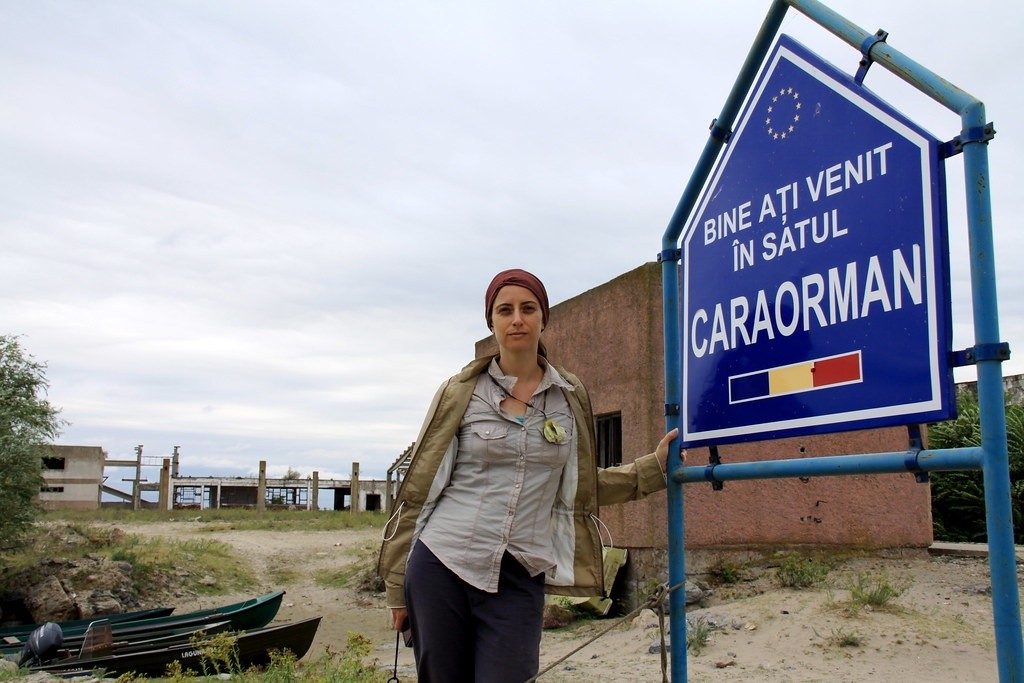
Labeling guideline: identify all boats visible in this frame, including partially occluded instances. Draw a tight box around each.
[0,615,324,678]
[0,607,177,636]
[0,591,286,655]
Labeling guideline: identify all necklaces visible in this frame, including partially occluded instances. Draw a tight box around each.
[484,371,566,444]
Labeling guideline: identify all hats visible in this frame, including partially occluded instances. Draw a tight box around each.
[485,269,550,333]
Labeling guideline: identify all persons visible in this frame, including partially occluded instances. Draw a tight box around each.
[374,269,687,682]
